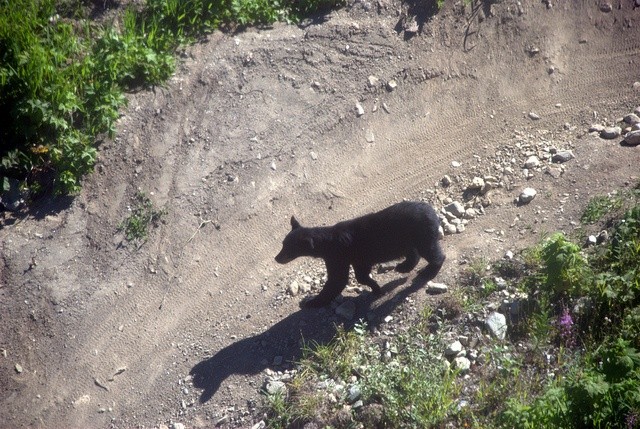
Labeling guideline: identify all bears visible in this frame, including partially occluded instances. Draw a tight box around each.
[274,202,446,307]
